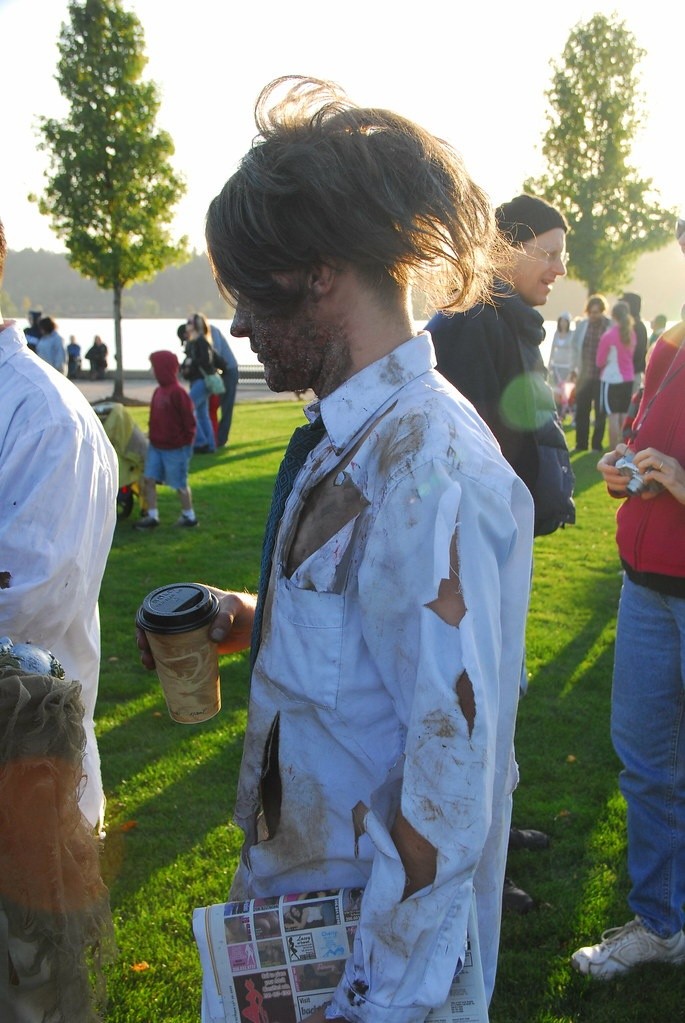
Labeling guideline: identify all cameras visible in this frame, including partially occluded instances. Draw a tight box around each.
[615,454,668,496]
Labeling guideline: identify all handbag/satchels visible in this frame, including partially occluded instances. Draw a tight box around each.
[205,372,227,395]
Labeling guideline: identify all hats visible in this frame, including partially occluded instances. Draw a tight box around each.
[177,325,186,347]
[618,292,642,304]
[494,195,569,246]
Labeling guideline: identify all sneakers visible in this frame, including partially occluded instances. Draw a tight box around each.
[570,915,684,984]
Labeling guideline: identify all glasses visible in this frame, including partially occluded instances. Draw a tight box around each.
[535,251,570,266]
[0,636,65,684]
[675,220,685,240]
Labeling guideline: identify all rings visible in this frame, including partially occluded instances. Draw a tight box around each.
[658,460,664,470]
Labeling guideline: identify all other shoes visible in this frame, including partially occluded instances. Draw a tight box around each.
[171,515,201,529]
[135,516,162,530]
[194,444,216,454]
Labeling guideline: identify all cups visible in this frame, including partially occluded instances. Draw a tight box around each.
[137,581,221,724]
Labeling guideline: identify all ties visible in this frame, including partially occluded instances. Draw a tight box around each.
[250,413,325,691]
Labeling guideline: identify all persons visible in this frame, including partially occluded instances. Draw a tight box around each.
[548,293,685,451]
[569,209,685,979]
[177,311,239,454]
[0,218,119,839]
[136,75,536,1023]
[424,193,577,915]
[348,887,364,911]
[134,350,198,528]
[23,307,107,380]
[284,903,335,929]
